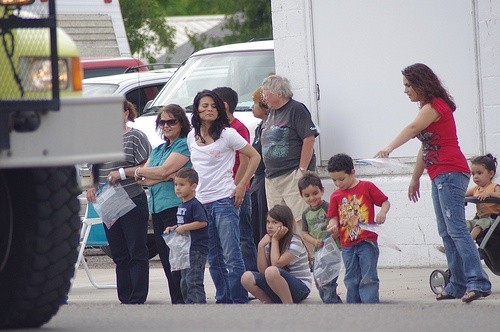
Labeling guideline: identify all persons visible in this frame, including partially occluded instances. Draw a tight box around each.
[260,75,319,263]
[212,87,259,299]
[373,63,492,302]
[241,204,312,304]
[87,100,152,304]
[327,153,390,303]
[437,154,500,253]
[187,90,261,304]
[134,104,193,304]
[250,88,268,252]
[163,168,208,304]
[298,171,344,304]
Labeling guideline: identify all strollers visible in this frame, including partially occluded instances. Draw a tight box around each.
[429,197,500,295]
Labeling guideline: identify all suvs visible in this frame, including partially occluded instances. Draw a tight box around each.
[0,0,127,330]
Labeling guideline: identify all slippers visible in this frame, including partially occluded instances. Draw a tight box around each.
[462,290,489,303]
[436,292,456,300]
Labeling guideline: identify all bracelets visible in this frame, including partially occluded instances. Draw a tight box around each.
[118,168,127,180]
[299,167,306,173]
[135,167,142,178]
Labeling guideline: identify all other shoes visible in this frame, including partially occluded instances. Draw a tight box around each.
[438,245,445,253]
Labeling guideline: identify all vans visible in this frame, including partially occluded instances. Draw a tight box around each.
[78,38,274,259]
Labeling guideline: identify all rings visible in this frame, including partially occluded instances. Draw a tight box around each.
[108,176,112,182]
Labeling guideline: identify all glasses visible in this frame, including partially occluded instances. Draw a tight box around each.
[159,118,177,126]
